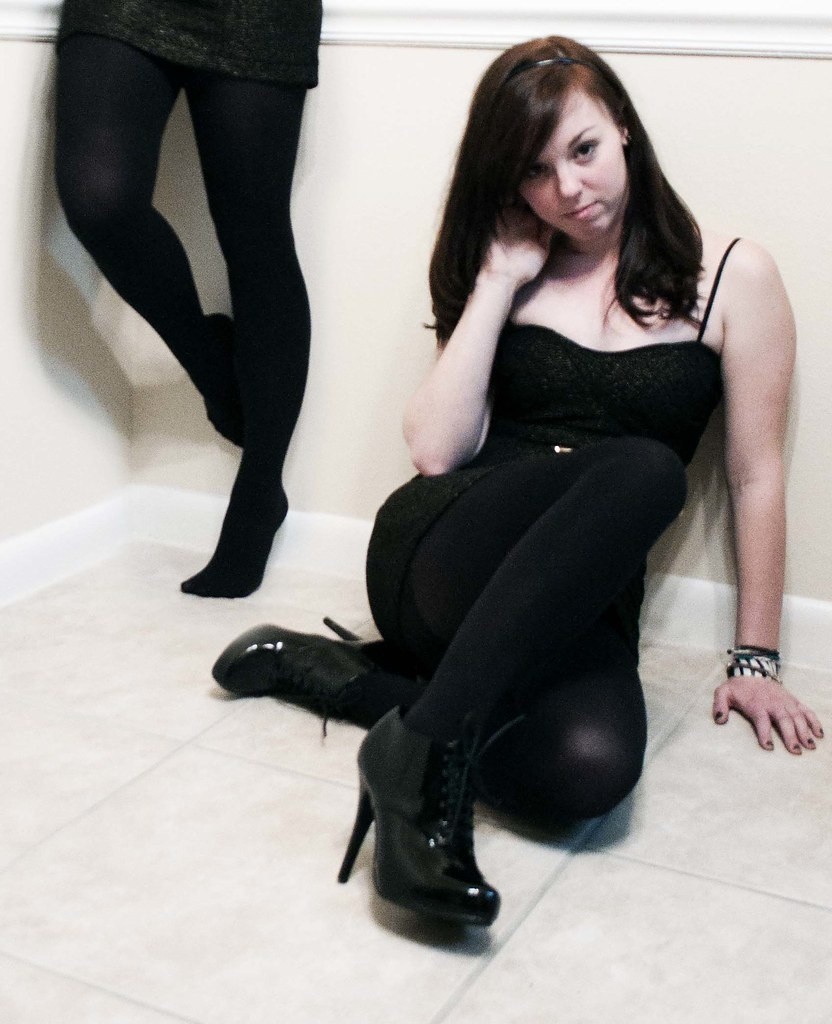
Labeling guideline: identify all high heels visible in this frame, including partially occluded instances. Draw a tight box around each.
[336,706,504,928]
[213,618,390,723]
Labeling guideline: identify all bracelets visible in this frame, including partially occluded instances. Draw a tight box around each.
[726,644,780,682]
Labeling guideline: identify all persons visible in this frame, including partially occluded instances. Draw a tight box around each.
[52,0,324,599]
[211,37,823,929]
